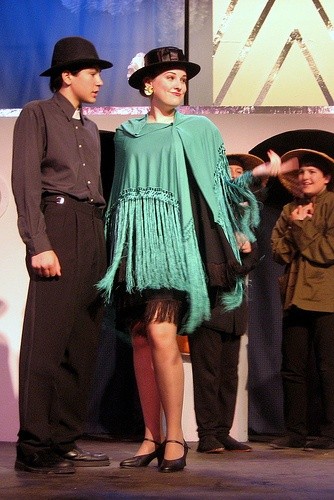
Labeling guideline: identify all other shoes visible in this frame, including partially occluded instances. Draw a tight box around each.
[196,435,224,453]
[301,437,334,451]
[268,434,302,449]
[217,434,253,452]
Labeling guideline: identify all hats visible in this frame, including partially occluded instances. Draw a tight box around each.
[278,149,334,198]
[226,153,269,194]
[131,44,203,90]
[41,37,113,74]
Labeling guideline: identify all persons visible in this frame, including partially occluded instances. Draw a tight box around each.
[95,46,334,473]
[11,37,112,473]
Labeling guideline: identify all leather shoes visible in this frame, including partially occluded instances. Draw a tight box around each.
[14,460,78,478]
[62,446,110,467]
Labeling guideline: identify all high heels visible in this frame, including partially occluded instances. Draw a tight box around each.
[120,437,166,469]
[158,439,191,473]
